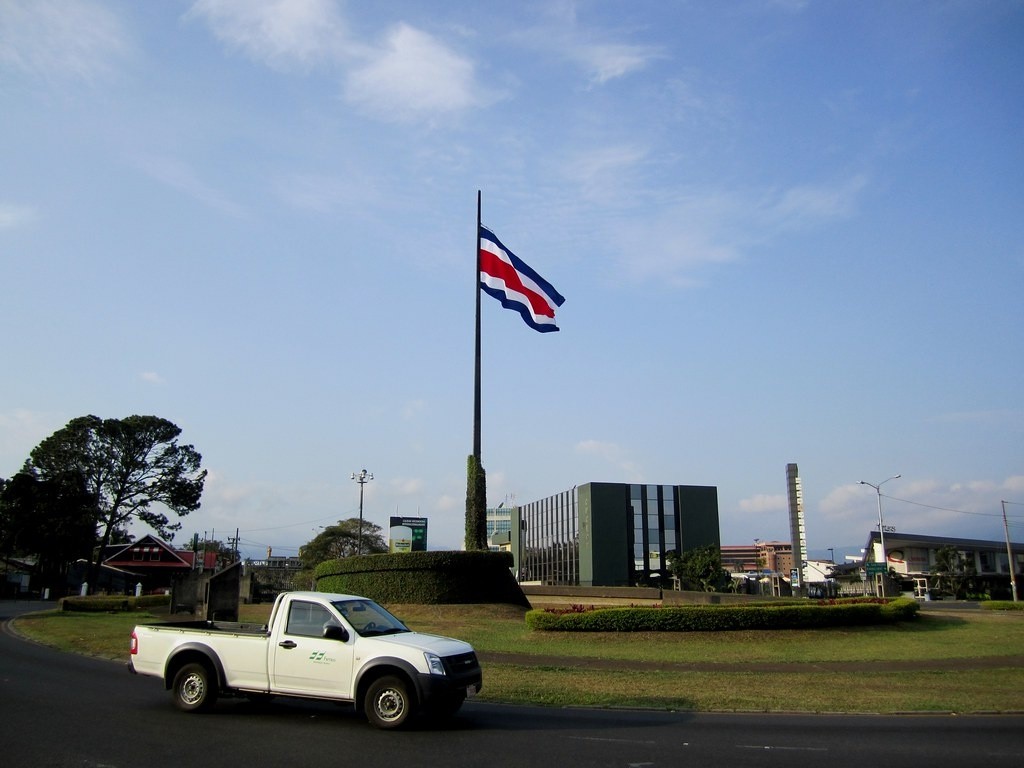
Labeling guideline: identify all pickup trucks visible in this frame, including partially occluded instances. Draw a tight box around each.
[128,591,482,731]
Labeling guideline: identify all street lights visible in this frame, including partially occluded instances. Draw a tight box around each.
[754,539,759,574]
[351,469,374,554]
[4,543,17,580]
[856,474,904,562]
[828,548,834,565]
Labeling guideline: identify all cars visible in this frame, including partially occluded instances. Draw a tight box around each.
[143,584,170,595]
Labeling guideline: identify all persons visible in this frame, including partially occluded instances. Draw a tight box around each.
[322,604,348,637]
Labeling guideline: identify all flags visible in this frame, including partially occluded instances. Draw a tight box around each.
[478,222,567,334]
